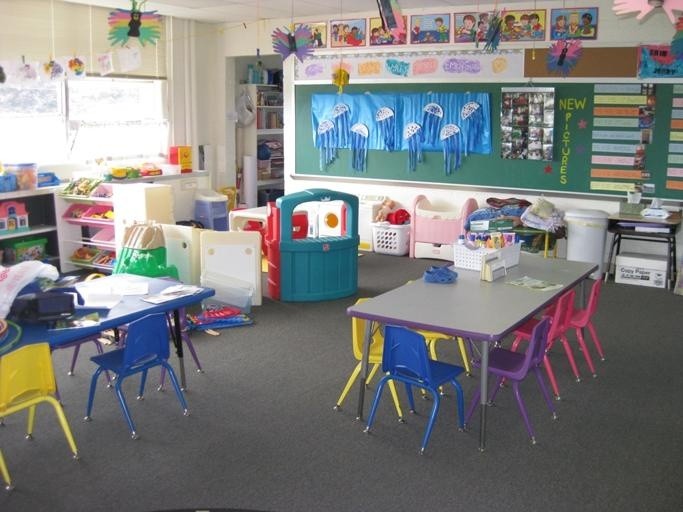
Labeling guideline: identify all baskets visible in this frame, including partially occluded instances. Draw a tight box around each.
[369,220,411,258]
[453,239,525,273]
[61,181,115,274]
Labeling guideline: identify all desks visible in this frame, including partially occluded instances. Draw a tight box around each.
[604,212,681,293]
[347,250,598,454]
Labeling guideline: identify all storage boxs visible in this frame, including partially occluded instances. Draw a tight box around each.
[615,251,669,288]
[62,178,116,269]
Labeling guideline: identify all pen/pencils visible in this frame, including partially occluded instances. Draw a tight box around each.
[458,234,512,249]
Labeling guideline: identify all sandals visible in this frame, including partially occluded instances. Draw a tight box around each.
[425,265,458,284]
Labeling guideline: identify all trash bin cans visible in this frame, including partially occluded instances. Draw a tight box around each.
[563,209,609,280]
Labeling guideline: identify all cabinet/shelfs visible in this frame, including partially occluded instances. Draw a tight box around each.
[157,172,213,222]
[194,192,228,231]
[234,83,283,207]
[58,182,172,277]
[409,196,475,261]
[0,181,81,273]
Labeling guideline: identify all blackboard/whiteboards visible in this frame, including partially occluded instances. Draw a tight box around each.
[290,82,683,206]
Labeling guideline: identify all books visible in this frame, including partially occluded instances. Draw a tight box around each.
[257,108,284,181]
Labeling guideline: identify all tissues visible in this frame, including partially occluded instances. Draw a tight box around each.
[641,198,672,219]
[619,192,645,218]
